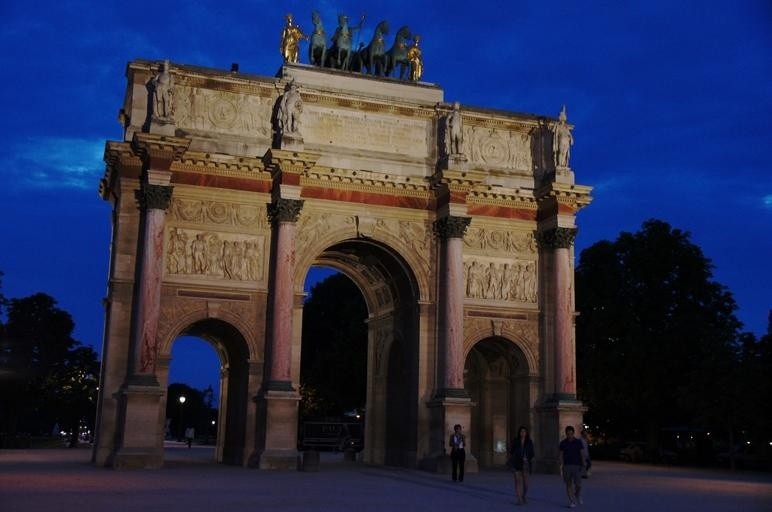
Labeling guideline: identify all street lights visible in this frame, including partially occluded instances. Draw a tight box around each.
[202,384,213,444]
[175,392,187,438]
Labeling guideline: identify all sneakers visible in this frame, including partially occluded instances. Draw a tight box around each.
[569,494,584,508]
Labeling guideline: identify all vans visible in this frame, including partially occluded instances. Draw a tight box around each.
[301,420,364,451]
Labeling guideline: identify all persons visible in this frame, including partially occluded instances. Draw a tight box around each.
[449,424,466,483]
[277,84,304,133]
[579,428,591,479]
[408,37,424,81]
[446,109,464,154]
[552,124,574,168]
[185,424,195,448]
[465,257,539,303]
[153,59,174,117]
[508,425,534,505]
[280,16,303,65]
[558,425,586,507]
[162,228,263,281]
